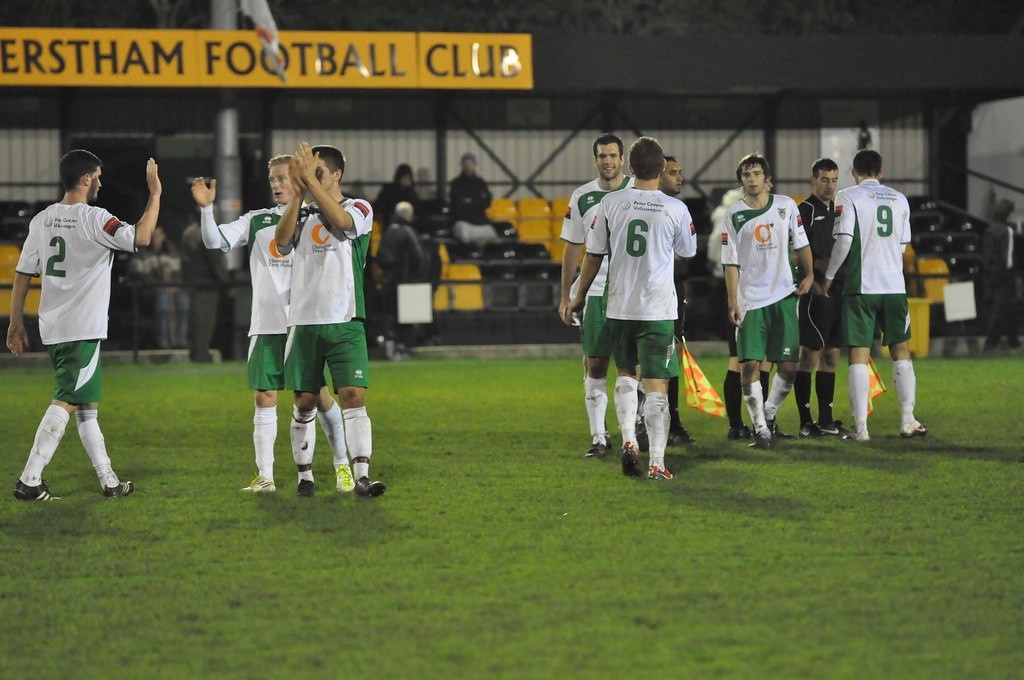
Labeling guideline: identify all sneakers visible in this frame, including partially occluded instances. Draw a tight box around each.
[103,478,134,499]
[354,476,386,497]
[900,420,928,438]
[799,420,851,440]
[841,429,871,441]
[334,464,355,493]
[648,466,676,481]
[13,478,62,501]
[616,441,643,478]
[727,425,754,439]
[240,472,276,495]
[768,423,796,440]
[748,425,772,450]
[297,479,315,497]
[636,426,697,451]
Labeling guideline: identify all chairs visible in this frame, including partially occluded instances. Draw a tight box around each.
[0,196,1024,319]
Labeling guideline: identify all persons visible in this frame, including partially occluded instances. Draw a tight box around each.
[362,153,493,361]
[558,134,928,478]
[192,155,355,492]
[981,198,1024,353]
[7,150,163,501]
[274,142,386,497]
[130,204,238,362]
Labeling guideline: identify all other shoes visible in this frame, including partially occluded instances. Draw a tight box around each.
[585,436,611,459]
[377,334,413,362]
